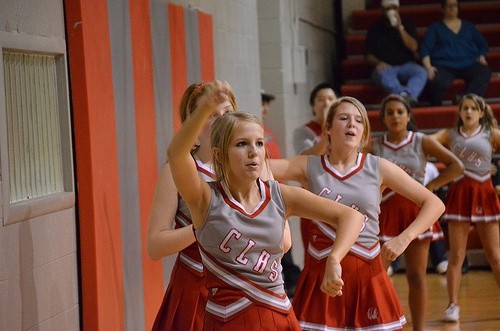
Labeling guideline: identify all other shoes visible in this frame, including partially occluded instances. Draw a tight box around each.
[435,260,449,274]
[401,91,418,105]
[387,265,398,277]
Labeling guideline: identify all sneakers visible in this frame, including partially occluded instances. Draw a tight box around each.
[445,303,461,322]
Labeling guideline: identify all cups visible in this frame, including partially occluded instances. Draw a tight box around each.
[387,9,398,26]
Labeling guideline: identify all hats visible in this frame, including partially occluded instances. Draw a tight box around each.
[381,0,399,8]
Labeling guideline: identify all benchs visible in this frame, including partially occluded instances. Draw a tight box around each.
[341,2,500,273]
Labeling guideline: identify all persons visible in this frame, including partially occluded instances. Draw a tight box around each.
[148,83,292,331]
[265,97,446,331]
[166,79,364,331]
[261,0,500,331]
